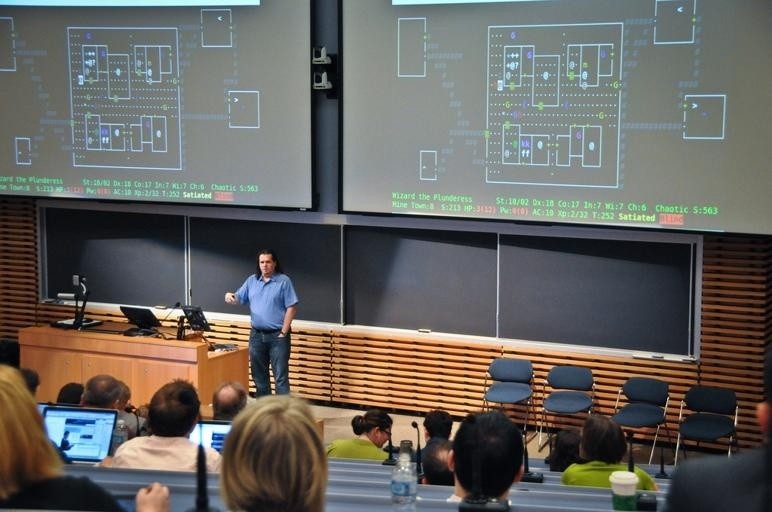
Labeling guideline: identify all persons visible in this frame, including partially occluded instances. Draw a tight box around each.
[18,369,146,456]
[561,416,659,490]
[225,248,299,396]
[326,409,393,461]
[220,395,328,512]
[447,411,525,512]
[661,348,772,512]
[111,378,222,474]
[549,429,589,471]
[212,382,248,420]
[61,431,71,450]
[0,363,171,512]
[420,437,455,486]
[411,409,452,462]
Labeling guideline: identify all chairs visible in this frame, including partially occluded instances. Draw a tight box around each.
[479,356,542,448]
[611,374,677,466]
[674,383,741,464]
[536,361,599,452]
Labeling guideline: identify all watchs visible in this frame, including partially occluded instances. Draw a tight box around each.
[280,331,288,337]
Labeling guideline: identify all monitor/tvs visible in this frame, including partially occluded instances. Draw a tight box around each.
[183,305,211,332]
[120,306,162,329]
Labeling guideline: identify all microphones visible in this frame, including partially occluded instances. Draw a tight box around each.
[156,302,180,331]
[125,407,140,437]
[412,421,424,480]
[519,431,543,482]
[626,429,657,512]
[382,432,398,466]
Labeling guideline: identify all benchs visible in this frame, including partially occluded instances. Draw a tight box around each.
[323,454,457,509]
[507,458,675,512]
[65,461,222,509]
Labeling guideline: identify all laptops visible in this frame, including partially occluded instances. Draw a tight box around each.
[37,402,80,418]
[42,406,118,467]
[189,420,232,458]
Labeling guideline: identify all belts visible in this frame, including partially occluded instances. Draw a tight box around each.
[251,328,280,334]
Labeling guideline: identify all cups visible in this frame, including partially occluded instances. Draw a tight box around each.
[639,492,656,512]
[608,469,640,512]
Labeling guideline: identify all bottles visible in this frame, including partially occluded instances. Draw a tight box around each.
[390,438,420,511]
